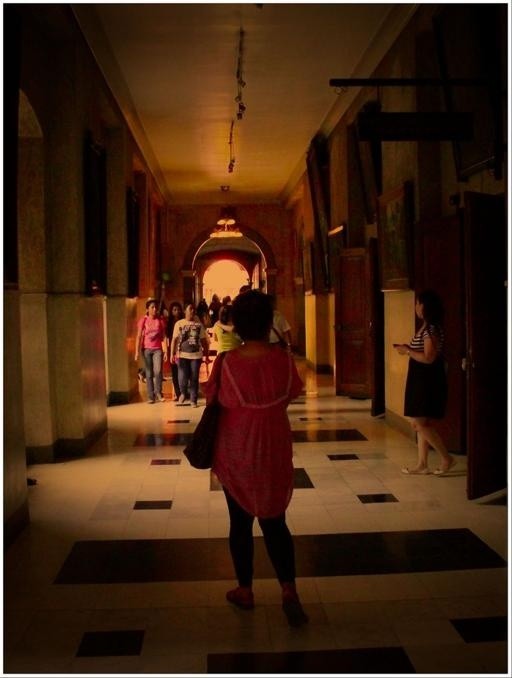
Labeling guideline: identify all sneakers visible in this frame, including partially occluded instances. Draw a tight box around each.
[173,393,185,405]
[191,402,197,408]
[147,394,166,403]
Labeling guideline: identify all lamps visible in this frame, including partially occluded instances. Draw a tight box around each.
[210,186,243,238]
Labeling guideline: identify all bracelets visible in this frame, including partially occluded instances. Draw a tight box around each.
[406,348,412,358]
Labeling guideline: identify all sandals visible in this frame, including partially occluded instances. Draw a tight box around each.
[434,456,459,477]
[403,466,430,476]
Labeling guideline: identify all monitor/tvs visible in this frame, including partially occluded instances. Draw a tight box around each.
[303,243,313,296]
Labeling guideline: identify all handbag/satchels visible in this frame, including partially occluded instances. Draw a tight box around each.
[182,349,230,470]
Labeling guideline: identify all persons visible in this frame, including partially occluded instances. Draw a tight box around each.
[204,288,314,630]
[134,282,300,408]
[390,278,458,478]
